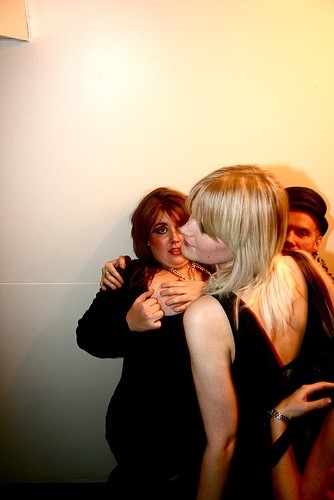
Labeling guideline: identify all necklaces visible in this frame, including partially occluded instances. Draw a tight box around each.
[160,261,198,281]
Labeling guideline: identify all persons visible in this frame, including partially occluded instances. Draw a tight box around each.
[76,187,219,500]
[281,187,328,256]
[269,381,334,500]
[178,164,334,500]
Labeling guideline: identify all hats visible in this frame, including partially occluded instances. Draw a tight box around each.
[283,186,329,237]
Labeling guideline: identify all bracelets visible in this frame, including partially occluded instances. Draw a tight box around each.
[270,409,291,425]
